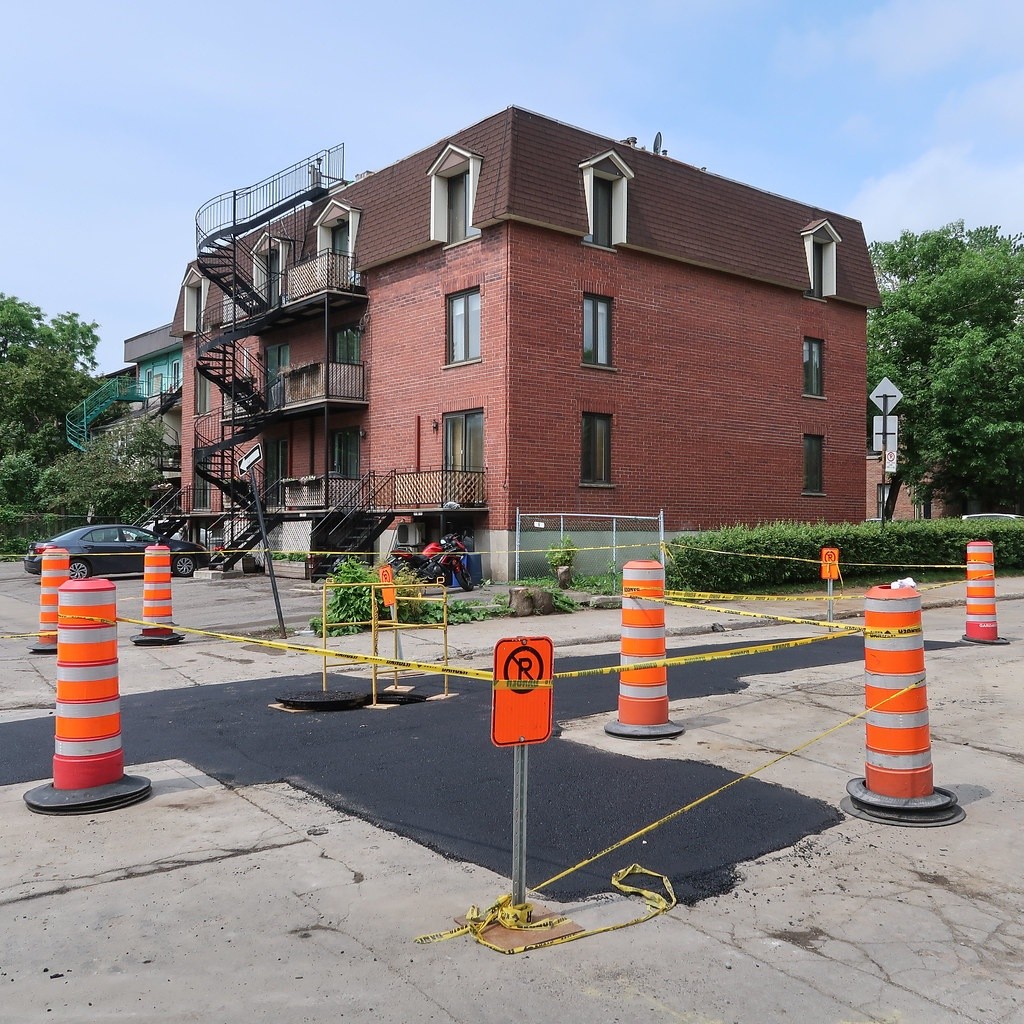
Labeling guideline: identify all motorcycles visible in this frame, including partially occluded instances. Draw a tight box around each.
[385,531,474,594]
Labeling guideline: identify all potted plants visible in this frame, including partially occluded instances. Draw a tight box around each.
[264,553,315,579]
[277,360,318,378]
[544,535,578,590]
[280,478,300,487]
[298,475,319,486]
[234,554,256,573]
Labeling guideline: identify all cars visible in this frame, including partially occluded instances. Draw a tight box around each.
[24,524,209,581]
[115,519,212,547]
[866,518,893,524]
[961,512,1024,525]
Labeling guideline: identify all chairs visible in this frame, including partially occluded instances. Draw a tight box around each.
[105,533,142,542]
